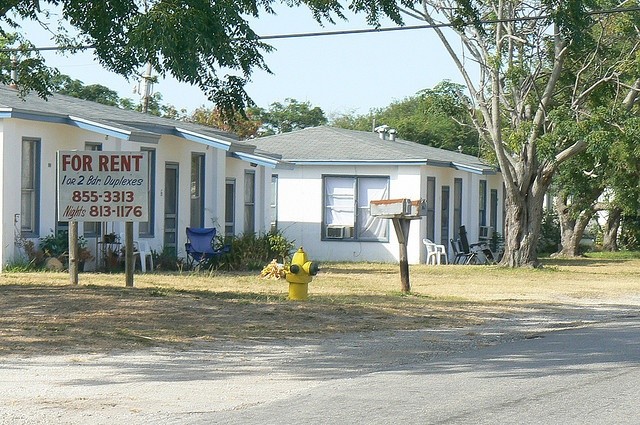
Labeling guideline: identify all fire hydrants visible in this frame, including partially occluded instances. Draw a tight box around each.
[285,246,319,302]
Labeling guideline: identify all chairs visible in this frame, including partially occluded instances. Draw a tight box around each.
[450,239,478,265]
[456,239,482,265]
[423,238,449,266]
[458,225,498,265]
[185,227,231,271]
[132,241,154,275]
